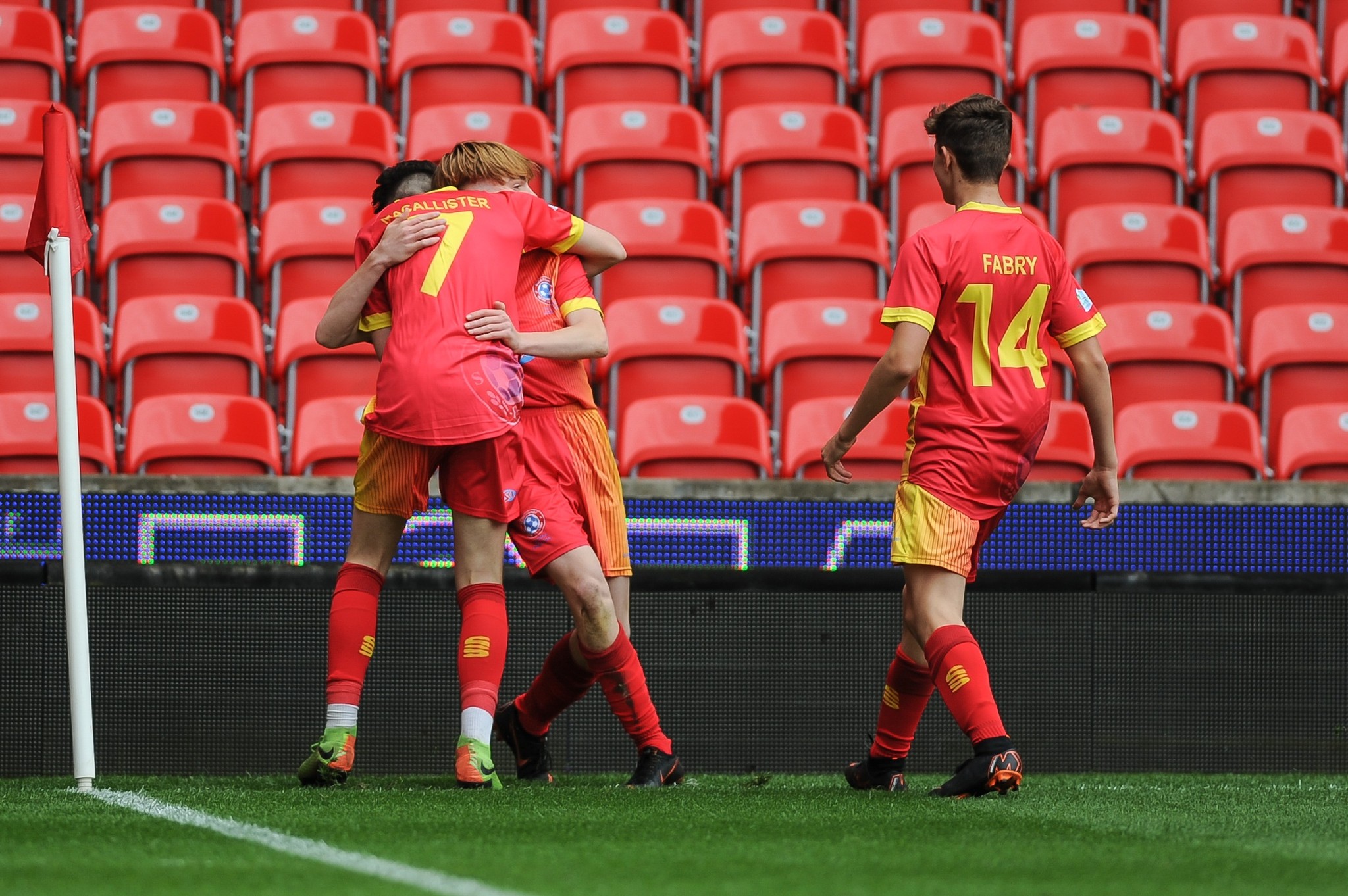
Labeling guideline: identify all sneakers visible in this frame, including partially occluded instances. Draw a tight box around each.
[455,736,503,790]
[296,725,357,787]
[627,746,685,790]
[491,700,553,784]
[927,747,1023,800]
[844,758,905,794]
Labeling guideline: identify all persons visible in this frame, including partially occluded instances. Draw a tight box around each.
[315,141,687,790]
[821,94,1120,795]
[296,160,602,790]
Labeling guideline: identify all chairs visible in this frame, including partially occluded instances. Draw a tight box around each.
[0,0,1348,482]
